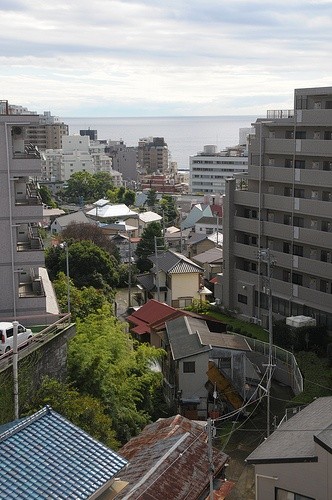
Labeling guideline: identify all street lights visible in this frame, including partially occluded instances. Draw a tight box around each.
[60,241,71,314]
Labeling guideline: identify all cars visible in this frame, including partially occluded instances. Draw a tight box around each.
[0,321,32,356]
